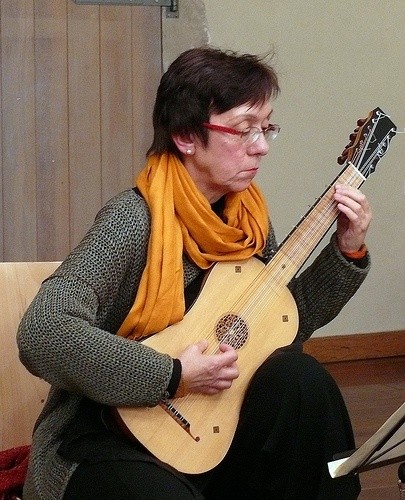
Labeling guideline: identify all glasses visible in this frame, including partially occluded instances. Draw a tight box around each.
[199,123,280,145]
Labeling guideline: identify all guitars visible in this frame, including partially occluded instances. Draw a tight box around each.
[115,106,397,483]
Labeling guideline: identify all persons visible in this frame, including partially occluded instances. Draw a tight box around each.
[16,48,374,500]
